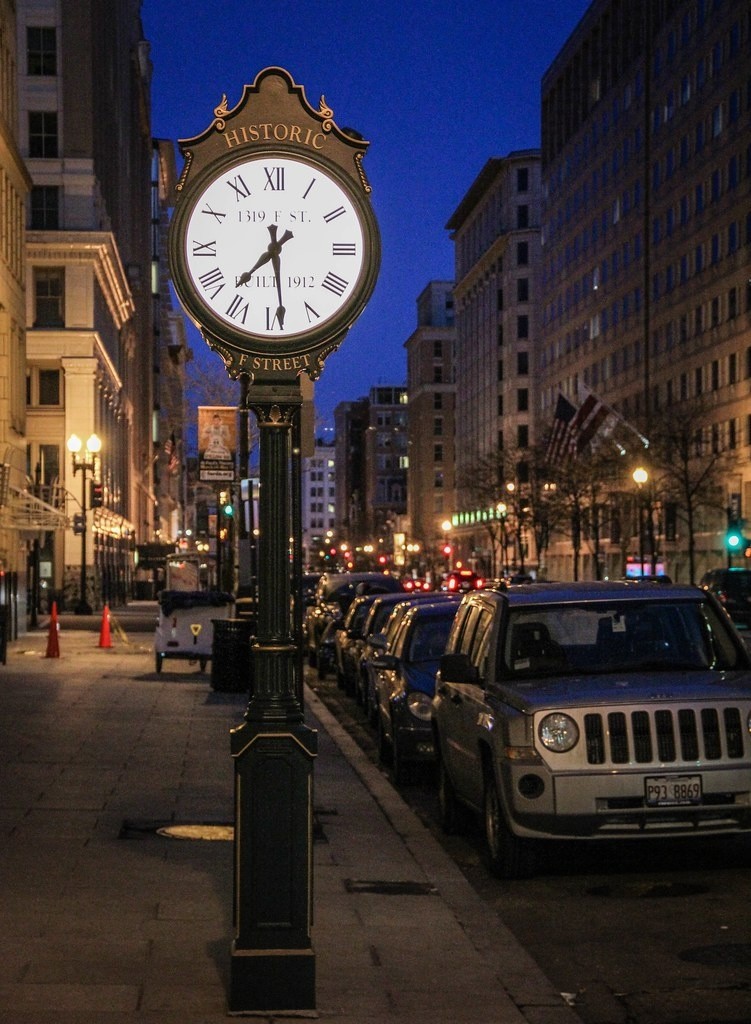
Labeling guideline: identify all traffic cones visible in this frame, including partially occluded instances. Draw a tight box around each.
[43,603,61,658]
[96,604,115,648]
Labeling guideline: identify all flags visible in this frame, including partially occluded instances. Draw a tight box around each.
[545,387,618,470]
[163,433,180,468]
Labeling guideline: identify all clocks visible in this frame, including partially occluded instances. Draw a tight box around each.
[167,66,380,405]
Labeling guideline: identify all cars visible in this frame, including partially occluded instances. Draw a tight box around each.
[318,592,464,722]
[442,571,485,596]
[405,576,431,592]
[370,599,461,789]
[290,571,327,629]
[304,573,400,666]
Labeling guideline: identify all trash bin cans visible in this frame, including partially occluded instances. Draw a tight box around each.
[210,617,253,693]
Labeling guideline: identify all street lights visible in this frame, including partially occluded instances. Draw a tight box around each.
[496,501,510,581]
[67,433,103,615]
[442,521,450,571]
[633,466,648,576]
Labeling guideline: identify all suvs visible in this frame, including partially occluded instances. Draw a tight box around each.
[701,567,751,646]
[431,578,751,878]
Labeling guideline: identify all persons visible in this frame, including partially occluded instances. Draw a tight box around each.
[156,568,165,590]
[201,415,232,446]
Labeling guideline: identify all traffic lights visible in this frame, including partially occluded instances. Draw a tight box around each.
[90,479,104,508]
[224,505,233,515]
[727,533,743,549]
